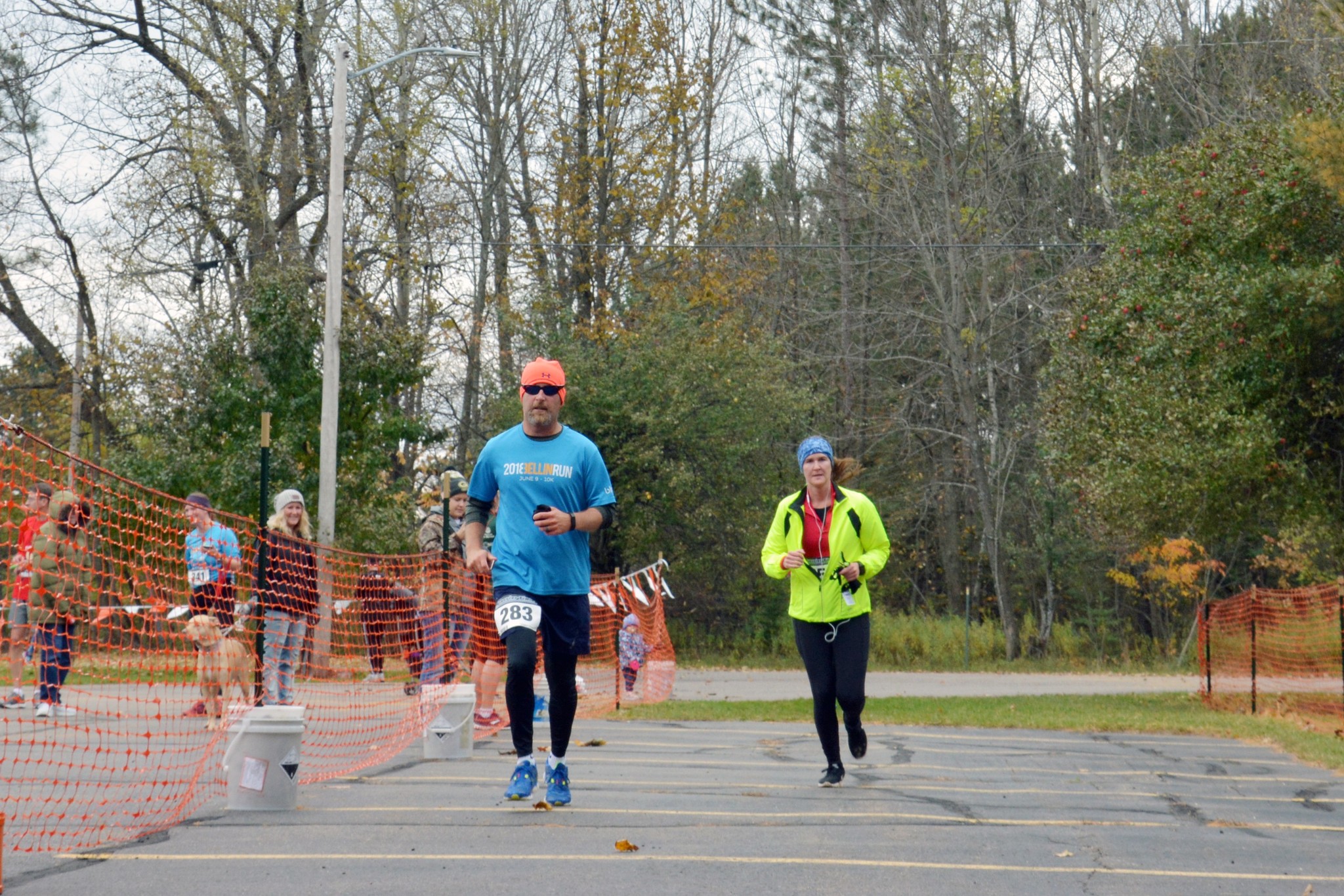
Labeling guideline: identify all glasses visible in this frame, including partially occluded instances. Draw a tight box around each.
[521,384,564,396]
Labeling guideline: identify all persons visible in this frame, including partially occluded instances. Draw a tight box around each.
[168,491,241,718]
[760,435,890,788]
[0,482,54,711]
[616,613,654,702]
[354,469,510,734]
[247,489,322,708]
[463,356,616,806]
[27,490,92,718]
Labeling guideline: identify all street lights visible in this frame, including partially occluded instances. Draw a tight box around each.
[307,40,481,680]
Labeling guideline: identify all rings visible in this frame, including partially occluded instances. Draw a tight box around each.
[546,526,550,533]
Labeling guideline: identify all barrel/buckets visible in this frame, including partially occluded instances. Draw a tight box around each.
[417,683,477,760]
[221,704,305,811]
[532,673,550,728]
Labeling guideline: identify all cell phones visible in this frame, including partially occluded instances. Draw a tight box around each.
[488,559,495,570]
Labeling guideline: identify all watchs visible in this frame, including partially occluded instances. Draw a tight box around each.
[854,561,866,576]
[568,512,576,532]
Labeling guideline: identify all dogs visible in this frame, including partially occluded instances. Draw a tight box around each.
[180,614,250,730]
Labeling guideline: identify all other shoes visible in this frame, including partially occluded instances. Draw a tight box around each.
[364,672,383,685]
[54,703,75,717]
[36,706,50,717]
[625,693,641,701]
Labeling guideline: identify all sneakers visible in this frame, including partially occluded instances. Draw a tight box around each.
[505,760,538,800]
[844,713,867,760]
[184,699,224,717]
[474,710,510,729]
[0,695,24,708]
[544,755,570,806]
[817,766,845,787]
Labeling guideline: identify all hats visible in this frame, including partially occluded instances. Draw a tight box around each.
[273,489,304,512]
[520,359,566,406]
[186,492,210,508]
[440,467,468,501]
[623,613,640,629]
